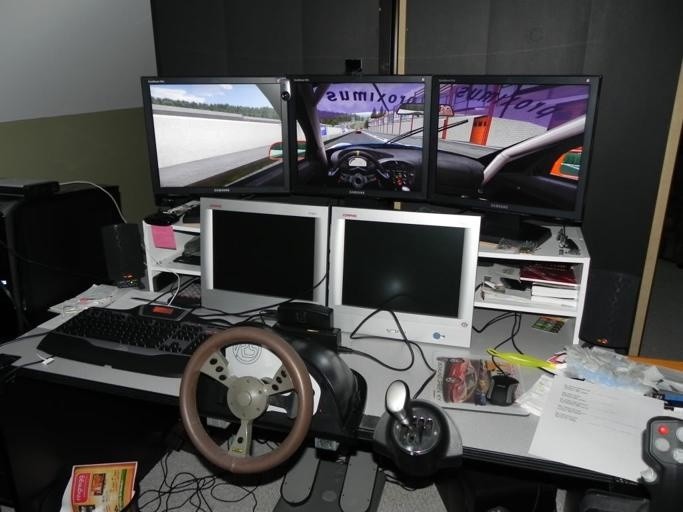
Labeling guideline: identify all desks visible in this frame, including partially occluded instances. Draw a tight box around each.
[0,282,683,511]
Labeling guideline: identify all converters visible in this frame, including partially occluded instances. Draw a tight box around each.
[2,173,61,199]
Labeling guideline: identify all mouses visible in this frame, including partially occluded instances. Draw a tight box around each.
[485,372,520,407]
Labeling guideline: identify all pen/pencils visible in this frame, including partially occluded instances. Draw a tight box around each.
[130,298,168,305]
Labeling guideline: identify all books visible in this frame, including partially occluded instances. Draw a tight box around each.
[481,261,578,309]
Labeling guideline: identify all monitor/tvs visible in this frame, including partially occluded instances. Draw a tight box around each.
[137,73,292,225]
[434,71,603,252]
[328,203,483,351]
[294,69,431,209]
[199,194,329,320]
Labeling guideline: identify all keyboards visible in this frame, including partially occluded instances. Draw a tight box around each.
[169,279,199,303]
[37,307,219,378]
